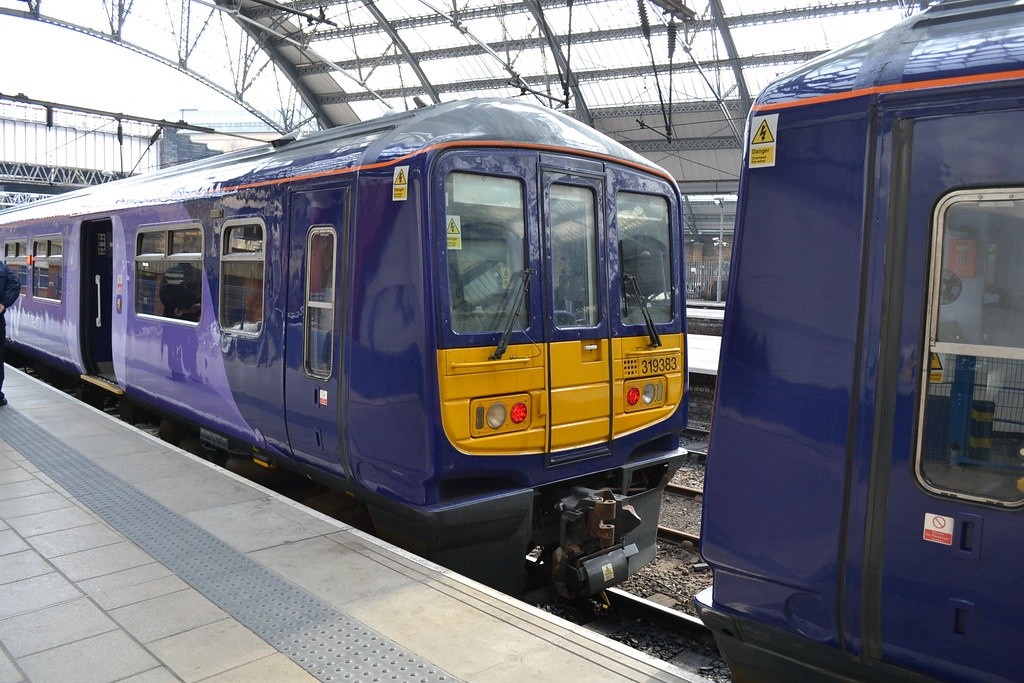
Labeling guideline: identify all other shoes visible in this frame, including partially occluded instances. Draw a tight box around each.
[0,392,7,406]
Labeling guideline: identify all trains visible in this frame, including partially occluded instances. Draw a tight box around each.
[0,94,688,599]
[688,1,1023,683]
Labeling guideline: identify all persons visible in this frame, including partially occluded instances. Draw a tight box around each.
[159,251,204,383]
[0,260,21,406]
